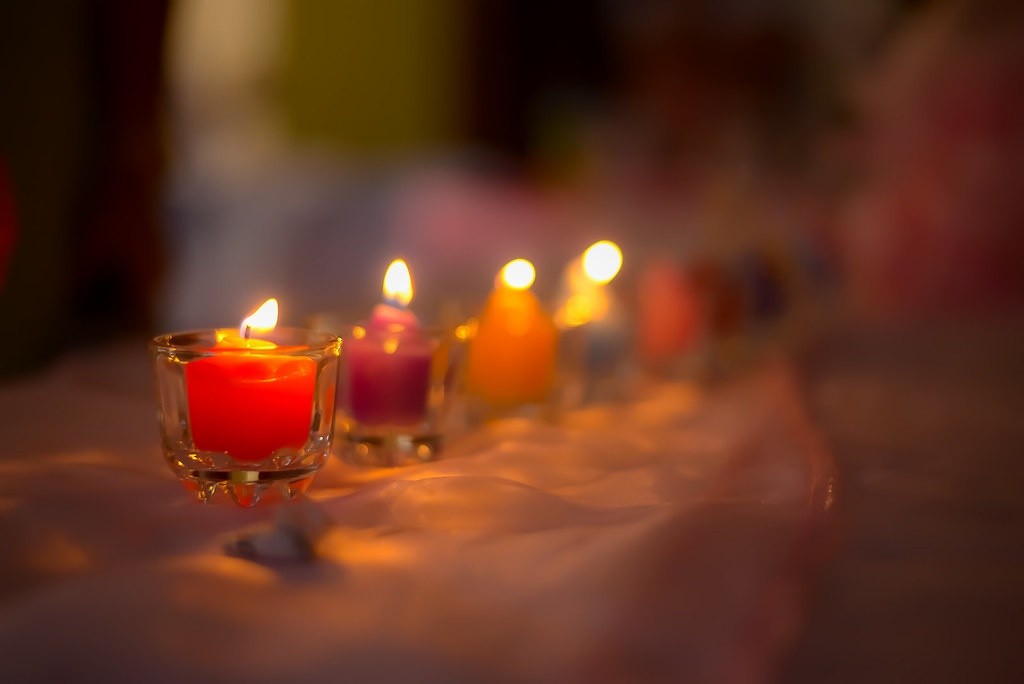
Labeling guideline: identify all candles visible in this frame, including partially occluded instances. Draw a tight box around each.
[343,259,437,427]
[555,240,636,377]
[183,298,319,467]
[466,256,556,406]
